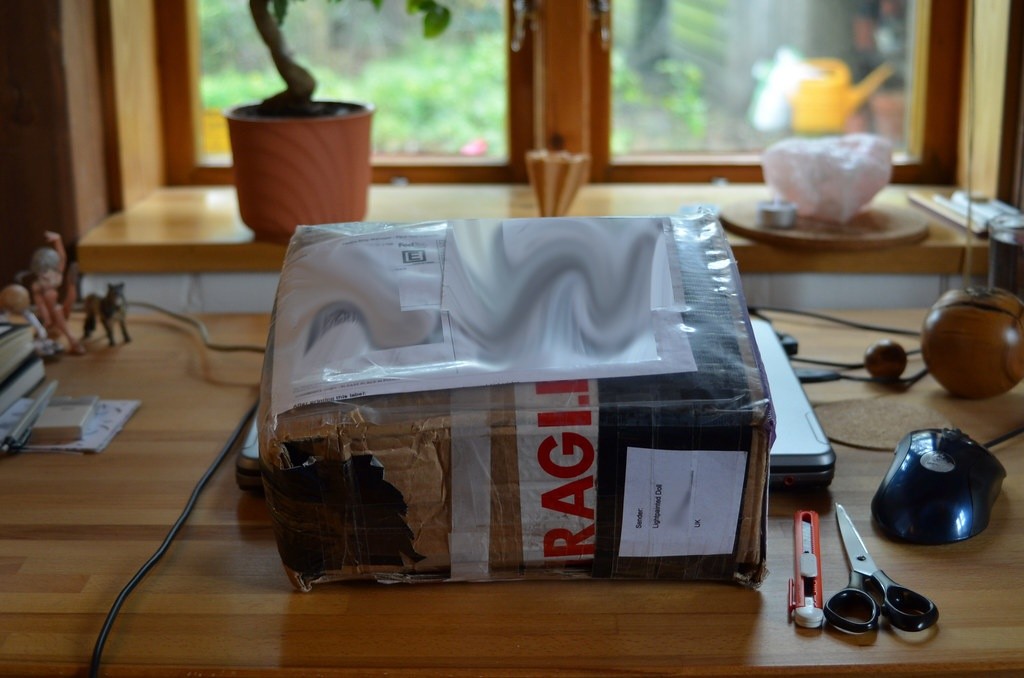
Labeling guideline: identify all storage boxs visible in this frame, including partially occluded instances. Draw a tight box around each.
[259,210,782,593]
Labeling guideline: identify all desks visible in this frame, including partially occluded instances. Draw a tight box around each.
[0,302,1024,677]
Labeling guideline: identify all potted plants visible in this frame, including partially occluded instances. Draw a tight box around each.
[222,1,451,243]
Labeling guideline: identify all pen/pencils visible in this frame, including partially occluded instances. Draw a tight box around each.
[0,379,63,455]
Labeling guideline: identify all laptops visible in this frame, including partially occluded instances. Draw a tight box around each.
[232,319,836,496]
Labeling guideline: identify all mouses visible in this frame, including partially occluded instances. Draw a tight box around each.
[871,427,1007,544]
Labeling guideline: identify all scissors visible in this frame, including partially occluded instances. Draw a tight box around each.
[824,499,939,638]
